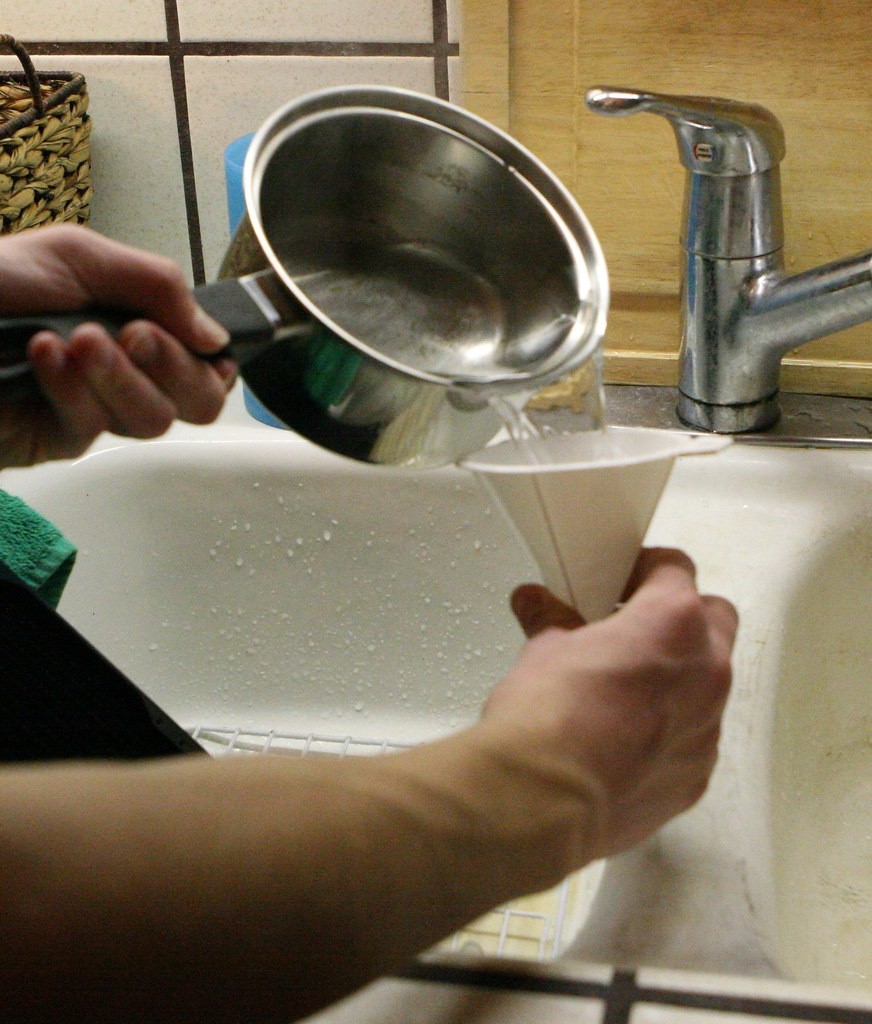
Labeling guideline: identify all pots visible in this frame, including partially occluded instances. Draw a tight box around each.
[0,85,609,470]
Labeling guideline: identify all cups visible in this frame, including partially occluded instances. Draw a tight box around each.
[224,132,291,431]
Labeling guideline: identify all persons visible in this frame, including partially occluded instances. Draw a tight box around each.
[0,223,739,1024]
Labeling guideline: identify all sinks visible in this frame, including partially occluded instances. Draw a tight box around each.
[709,428,871,1023]
[1,405,694,972]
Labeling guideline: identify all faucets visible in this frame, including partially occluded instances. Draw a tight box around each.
[582,84,871,435]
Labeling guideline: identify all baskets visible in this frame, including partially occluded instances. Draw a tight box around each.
[0,32,93,235]
